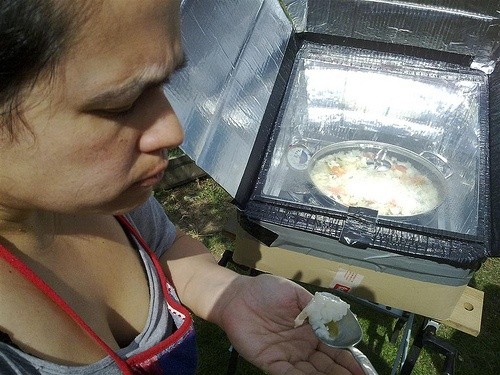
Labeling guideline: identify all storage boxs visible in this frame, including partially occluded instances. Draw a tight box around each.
[166,1,487,278]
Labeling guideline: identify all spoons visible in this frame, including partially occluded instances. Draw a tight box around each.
[309,292,377,375]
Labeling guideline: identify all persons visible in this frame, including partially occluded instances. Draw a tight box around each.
[0,0,367,375]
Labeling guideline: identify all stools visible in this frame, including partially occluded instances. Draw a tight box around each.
[222,211,485,375]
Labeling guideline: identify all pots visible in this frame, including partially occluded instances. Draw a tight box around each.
[285,140,455,225]
